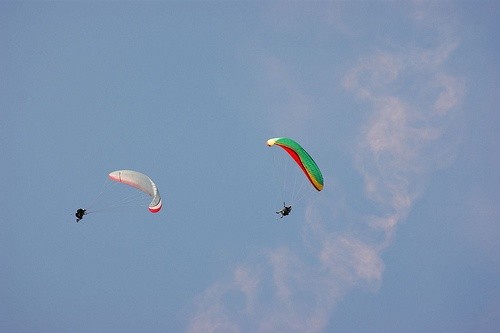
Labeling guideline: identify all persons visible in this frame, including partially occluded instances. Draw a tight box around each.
[75,208,86,222]
[276,201,291,218]
